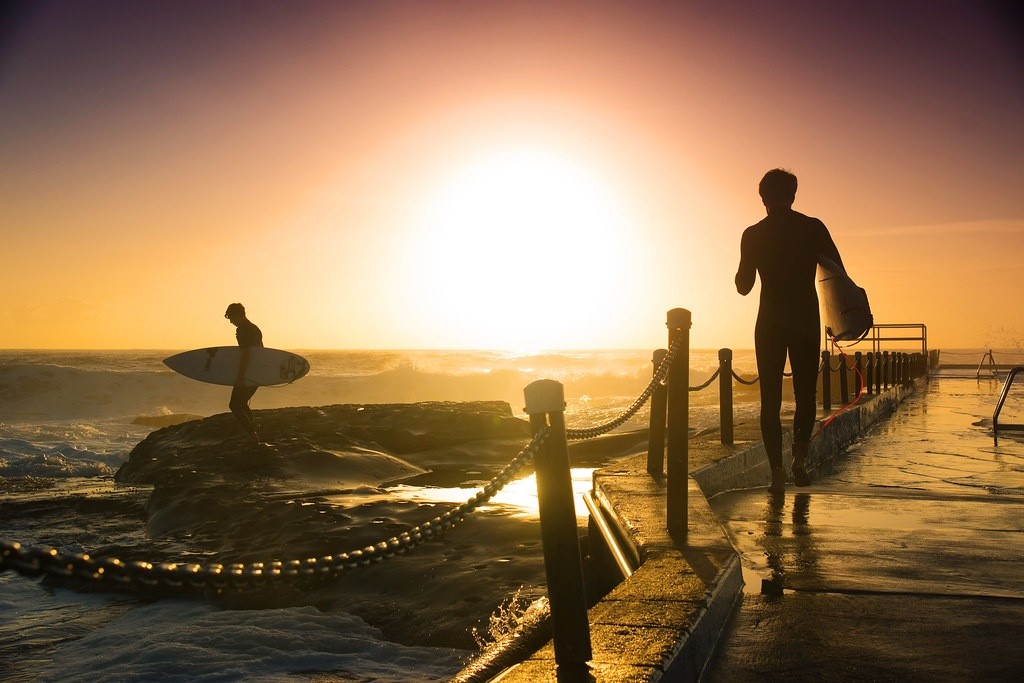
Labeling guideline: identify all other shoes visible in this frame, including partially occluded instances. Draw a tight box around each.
[768,481,785,493]
[792,458,812,488]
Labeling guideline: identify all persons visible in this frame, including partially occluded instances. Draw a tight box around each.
[225,303,264,444]
[734,168,846,494]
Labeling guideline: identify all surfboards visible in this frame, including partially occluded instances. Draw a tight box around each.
[162,345,310,390]
[815,249,874,342]
